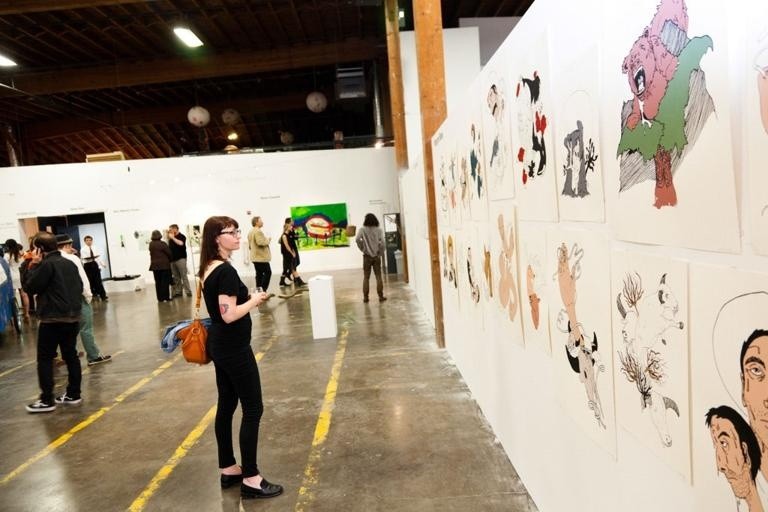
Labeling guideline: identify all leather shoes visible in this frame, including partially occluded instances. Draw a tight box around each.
[239,477,285,499]
[218,465,244,489]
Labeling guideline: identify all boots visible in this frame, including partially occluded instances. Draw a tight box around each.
[294,276,308,286]
[279,276,292,287]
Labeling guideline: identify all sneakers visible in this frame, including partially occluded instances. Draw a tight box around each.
[24,399,56,413]
[155,290,195,303]
[86,353,113,366]
[53,393,82,404]
[363,293,370,303]
[285,272,293,281]
[377,291,387,302]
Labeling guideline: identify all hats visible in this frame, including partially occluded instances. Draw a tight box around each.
[150,230,162,239]
[55,233,73,246]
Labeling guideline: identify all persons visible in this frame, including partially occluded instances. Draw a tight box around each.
[285,218,293,281]
[0,231,113,414]
[167,224,192,297]
[247,215,272,292]
[704,405,765,511]
[355,212,387,302]
[148,230,173,302]
[739,329,768,484]
[192,215,285,499]
[279,223,308,286]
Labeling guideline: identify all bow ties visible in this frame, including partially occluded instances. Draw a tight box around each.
[89,247,95,262]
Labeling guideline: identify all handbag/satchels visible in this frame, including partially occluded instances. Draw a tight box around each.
[177,276,214,365]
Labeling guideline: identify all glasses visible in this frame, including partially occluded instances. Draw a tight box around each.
[219,228,243,239]
